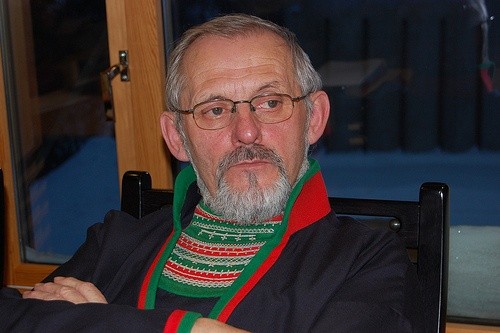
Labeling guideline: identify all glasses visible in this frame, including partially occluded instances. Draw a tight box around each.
[174,86,314,130]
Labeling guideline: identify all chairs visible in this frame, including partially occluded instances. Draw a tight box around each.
[120,169,449,331]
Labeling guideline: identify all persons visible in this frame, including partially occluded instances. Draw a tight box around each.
[444,0,491,148]
[1,13,416,332]
